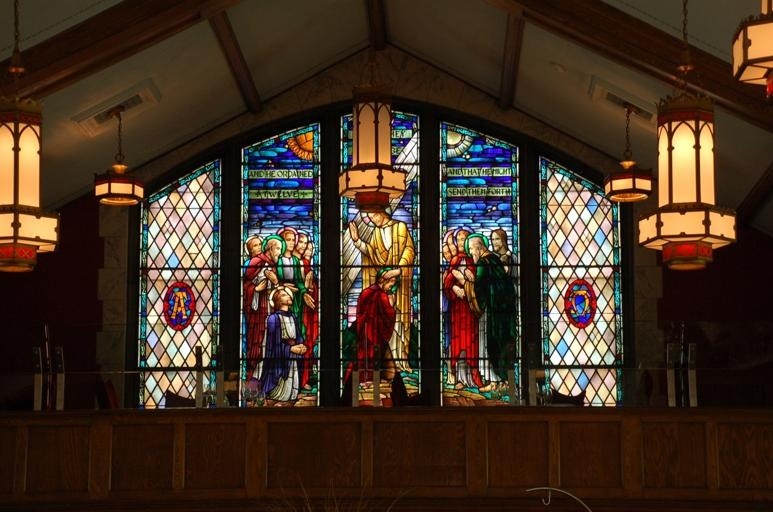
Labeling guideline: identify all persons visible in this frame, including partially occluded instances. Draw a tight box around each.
[442,229,518,392]
[349,207,416,381]
[244,226,318,401]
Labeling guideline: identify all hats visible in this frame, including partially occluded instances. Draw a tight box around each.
[355,192,390,213]
[269,287,293,308]
[244,227,315,258]
[443,227,488,258]
[376,268,401,295]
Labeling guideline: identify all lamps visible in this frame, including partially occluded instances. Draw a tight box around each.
[730,1,772,99]
[0,0,60,274]
[338,41,408,211]
[639,2,739,271]
[604,105,654,206]
[93,107,146,208]
